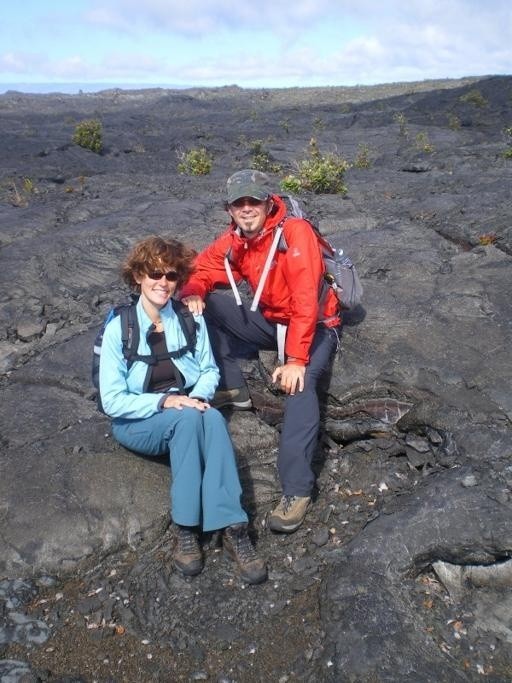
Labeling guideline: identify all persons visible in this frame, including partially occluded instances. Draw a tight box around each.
[178,169,343,532]
[96,236,268,585]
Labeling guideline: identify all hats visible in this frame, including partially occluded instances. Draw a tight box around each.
[226,169,272,206]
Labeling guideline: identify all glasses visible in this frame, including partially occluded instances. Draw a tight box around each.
[145,268,180,281]
[232,198,261,206]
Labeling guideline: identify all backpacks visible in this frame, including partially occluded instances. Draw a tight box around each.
[92,301,197,389]
[274,213,364,310]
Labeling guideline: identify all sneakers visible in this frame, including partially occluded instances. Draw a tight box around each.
[210,387,252,408]
[172,521,203,575]
[223,522,266,584]
[270,494,313,533]
